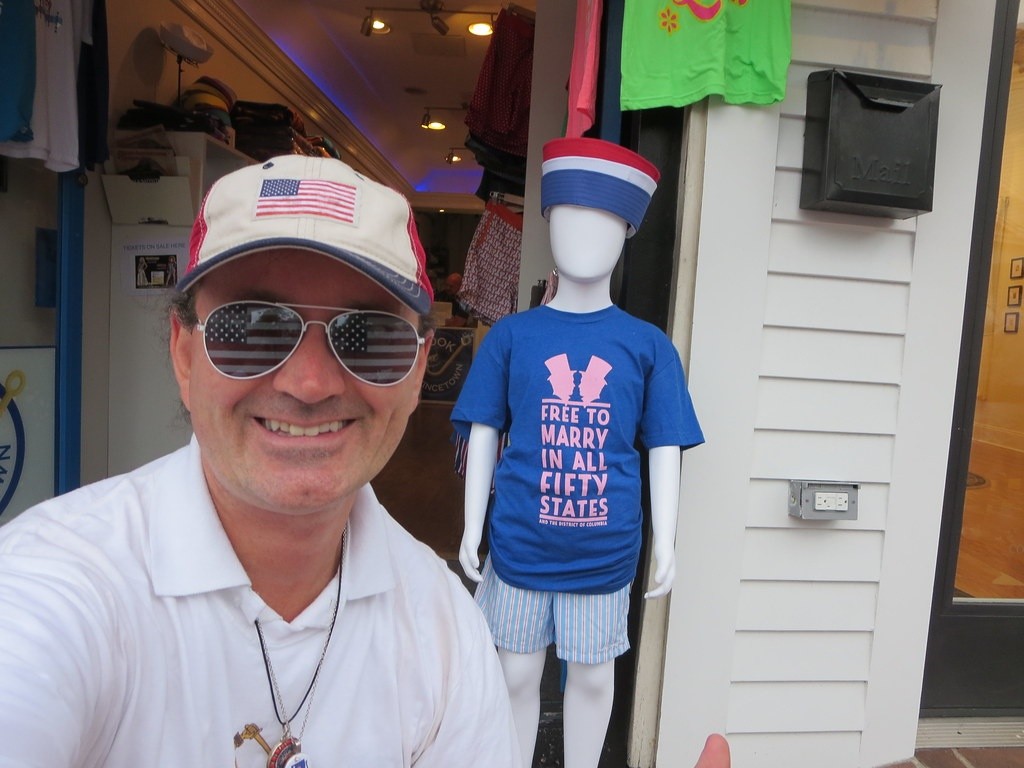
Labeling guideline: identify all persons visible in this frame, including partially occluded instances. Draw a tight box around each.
[449,137,706,768]
[0,154,731,768]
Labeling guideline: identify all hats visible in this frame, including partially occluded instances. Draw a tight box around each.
[541,135,660,237]
[160,20,214,63]
[179,77,237,126]
[175,155,435,313]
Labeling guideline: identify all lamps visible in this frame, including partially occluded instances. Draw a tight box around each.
[446,147,470,165]
[360,6,498,37]
[422,106,432,129]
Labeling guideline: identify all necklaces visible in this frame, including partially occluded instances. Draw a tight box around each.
[253,528,346,768]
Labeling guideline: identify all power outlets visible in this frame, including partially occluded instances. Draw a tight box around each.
[814,492,848,511]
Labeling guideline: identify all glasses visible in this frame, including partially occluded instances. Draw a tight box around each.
[197,300,427,387]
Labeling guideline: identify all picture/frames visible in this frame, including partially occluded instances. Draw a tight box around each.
[1003,256,1024,333]
